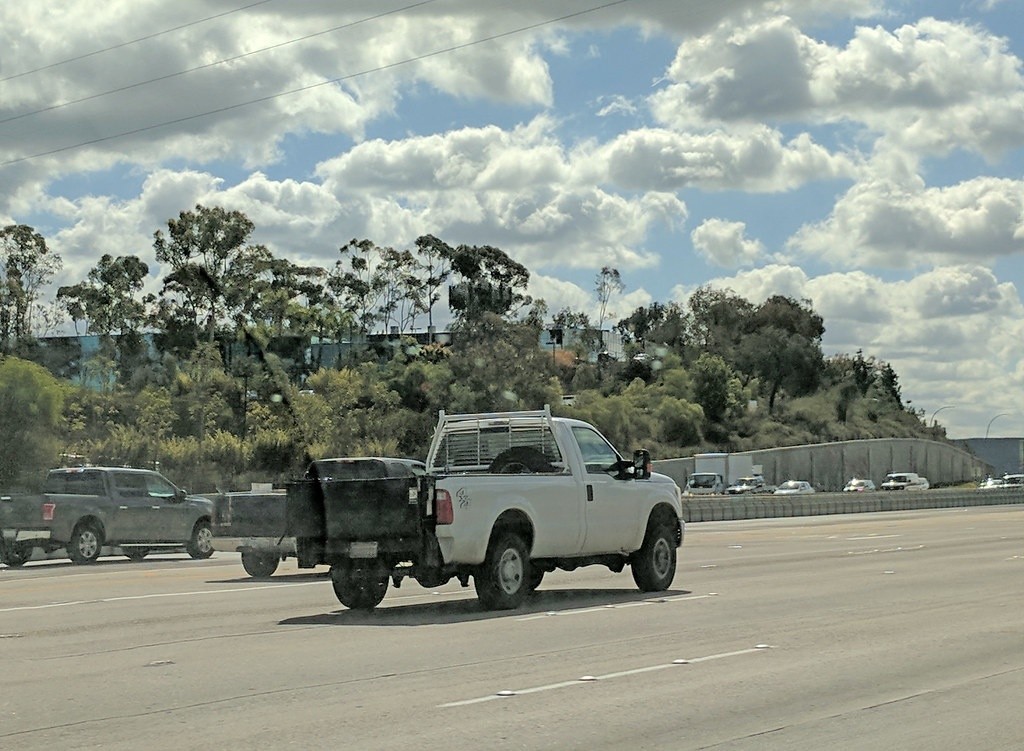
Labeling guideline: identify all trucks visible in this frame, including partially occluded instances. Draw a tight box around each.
[881,473,929,490]
[687,471,778,496]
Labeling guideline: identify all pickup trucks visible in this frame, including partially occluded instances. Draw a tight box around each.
[0,465,214,567]
[213,457,424,576]
[283,405,684,611]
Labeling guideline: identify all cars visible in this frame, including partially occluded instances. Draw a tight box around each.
[844,480,876,492]
[979,474,1024,488]
[773,480,815,494]
